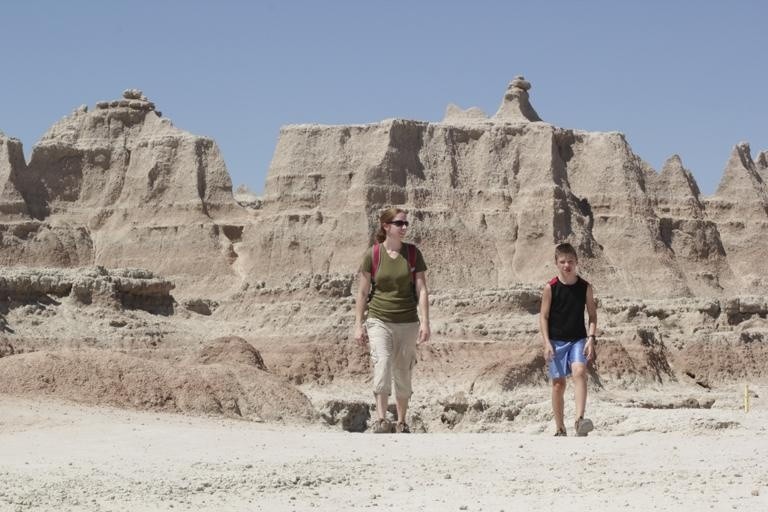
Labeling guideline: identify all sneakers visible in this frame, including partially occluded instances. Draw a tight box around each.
[555,429,568,436]
[374,419,392,433]
[575,419,594,437]
[392,423,410,433]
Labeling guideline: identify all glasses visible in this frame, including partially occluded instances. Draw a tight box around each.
[386,220,409,227]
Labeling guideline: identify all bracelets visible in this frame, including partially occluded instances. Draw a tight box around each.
[587,334,598,346]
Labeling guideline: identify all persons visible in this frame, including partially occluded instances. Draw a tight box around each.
[538,241,599,437]
[351,205,431,433]
